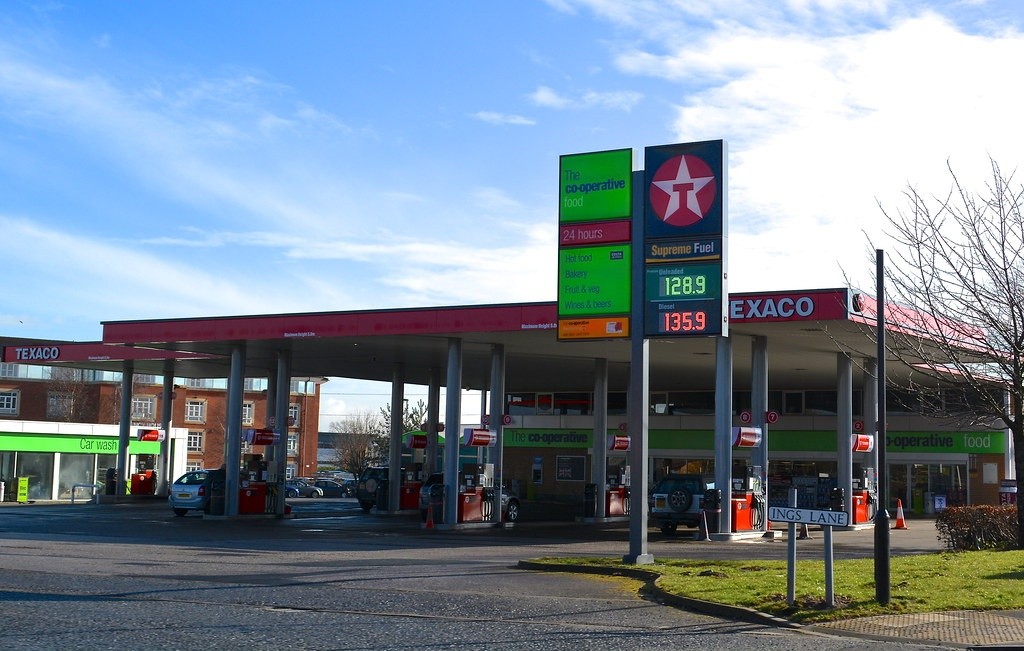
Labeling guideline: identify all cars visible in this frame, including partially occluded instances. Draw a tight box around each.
[341,480,359,497]
[314,480,351,498]
[286,480,323,498]
[285,484,299,498]
[168,470,210,516]
[418,471,521,522]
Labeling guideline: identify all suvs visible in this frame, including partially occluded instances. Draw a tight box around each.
[649,473,716,535]
[355,466,405,510]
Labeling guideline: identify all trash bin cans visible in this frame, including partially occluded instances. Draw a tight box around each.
[429,485,446,523]
[105,468,117,494]
[583,482,599,518]
[376,479,389,511]
[209,479,225,515]
[703,489,722,533]
[829,487,845,512]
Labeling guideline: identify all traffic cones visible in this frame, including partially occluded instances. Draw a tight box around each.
[696,511,712,542]
[424,503,435,529]
[796,523,813,540]
[890,499,910,530]
